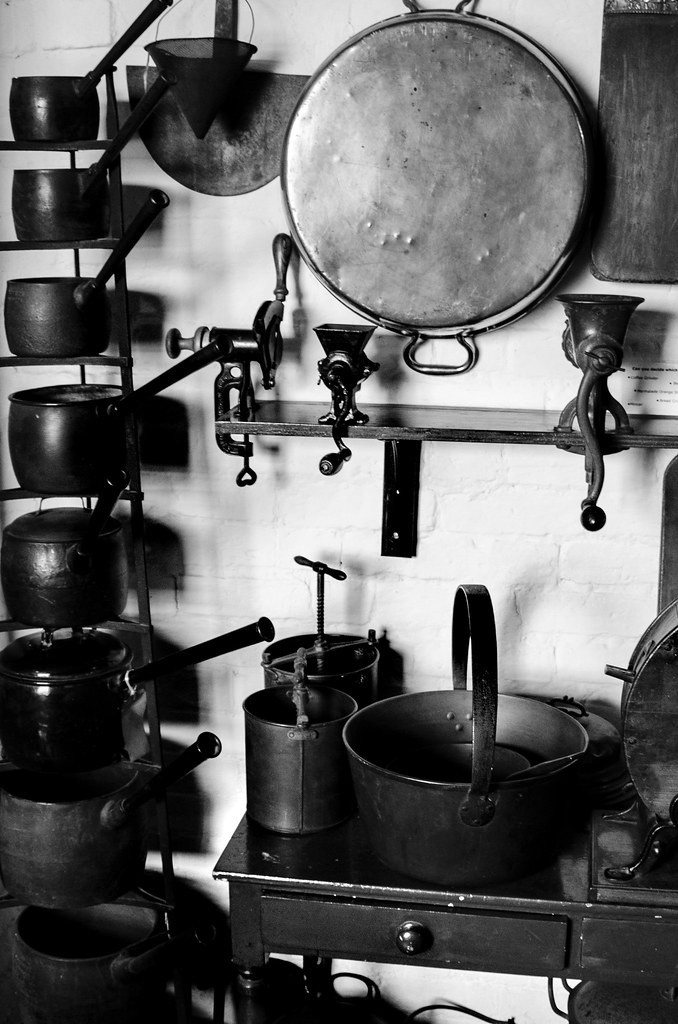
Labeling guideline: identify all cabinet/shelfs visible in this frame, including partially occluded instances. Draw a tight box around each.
[0,65,188,1024]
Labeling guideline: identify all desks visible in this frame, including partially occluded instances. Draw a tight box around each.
[214,812,678,1024]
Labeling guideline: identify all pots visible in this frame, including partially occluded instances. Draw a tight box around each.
[12,70,178,241]
[9,0,174,142]
[343,584,589,887]
[0,468,129,627]
[0,617,285,770]
[12,897,217,1024]
[4,189,170,356]
[0,732,222,909]
[8,336,234,492]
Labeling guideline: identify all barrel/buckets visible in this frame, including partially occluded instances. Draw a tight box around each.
[261,634,380,708]
[242,683,359,835]
